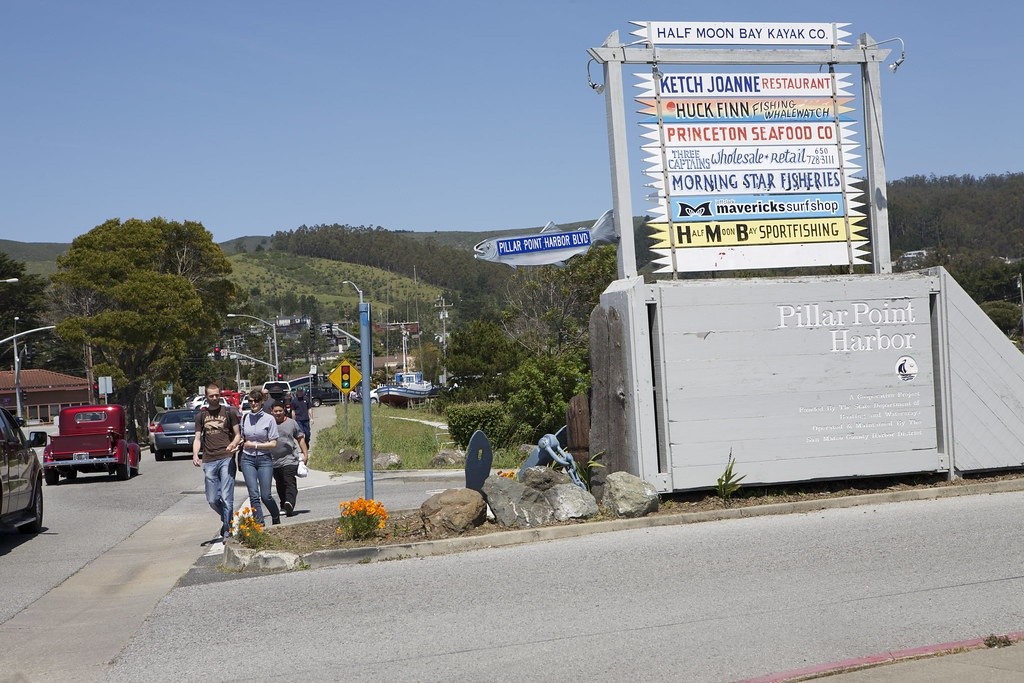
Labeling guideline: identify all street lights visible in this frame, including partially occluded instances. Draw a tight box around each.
[342,281,363,303]
[227,314,279,380]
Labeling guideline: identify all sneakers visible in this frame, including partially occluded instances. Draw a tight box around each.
[223,534,233,545]
[284,501,293,517]
[220,524,224,536]
[279,509,286,515]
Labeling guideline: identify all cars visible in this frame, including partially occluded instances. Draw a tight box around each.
[154,409,204,460]
[240,395,249,400]
[186,395,205,409]
[43,405,141,484]
[148,410,167,453]
[262,381,291,393]
[0,407,47,543]
[370,389,379,404]
[283,398,291,417]
[200,397,230,409]
[239,400,252,419]
[310,382,349,407]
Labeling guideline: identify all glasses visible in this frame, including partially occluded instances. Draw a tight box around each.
[248,399,262,403]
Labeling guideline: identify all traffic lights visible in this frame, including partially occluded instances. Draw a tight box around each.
[214,347,220,359]
[278,373,283,381]
[326,322,332,338]
[310,325,315,338]
[93,382,98,398]
[328,359,362,394]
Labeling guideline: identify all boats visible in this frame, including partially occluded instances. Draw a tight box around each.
[374,324,435,407]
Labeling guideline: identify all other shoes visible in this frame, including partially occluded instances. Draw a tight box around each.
[272,519,280,525]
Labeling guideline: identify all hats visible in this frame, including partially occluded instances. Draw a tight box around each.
[297,387,304,392]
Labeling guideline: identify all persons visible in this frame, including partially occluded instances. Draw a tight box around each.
[240,389,281,527]
[291,386,314,453]
[262,389,275,414]
[270,402,308,518]
[194,383,241,544]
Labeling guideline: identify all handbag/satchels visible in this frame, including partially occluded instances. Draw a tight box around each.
[238,447,244,472]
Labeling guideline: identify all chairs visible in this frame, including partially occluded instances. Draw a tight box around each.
[167,414,180,422]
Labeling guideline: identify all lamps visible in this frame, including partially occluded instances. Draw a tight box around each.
[587,57,605,94]
[631,40,663,80]
[856,37,905,73]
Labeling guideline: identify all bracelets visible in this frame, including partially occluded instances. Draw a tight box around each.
[254,442,258,450]
